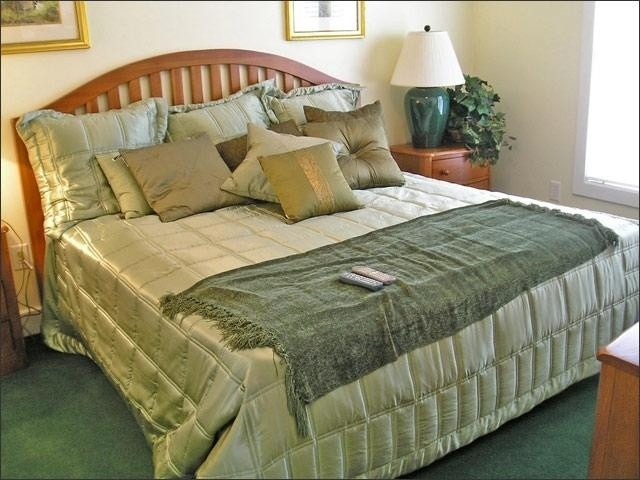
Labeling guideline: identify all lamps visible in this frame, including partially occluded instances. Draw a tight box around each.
[391,24,465,147]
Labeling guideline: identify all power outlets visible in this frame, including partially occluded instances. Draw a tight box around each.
[11,244,34,273]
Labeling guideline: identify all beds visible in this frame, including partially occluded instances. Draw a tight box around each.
[11,48,640,480]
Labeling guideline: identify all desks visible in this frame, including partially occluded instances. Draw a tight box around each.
[587,321,639,479]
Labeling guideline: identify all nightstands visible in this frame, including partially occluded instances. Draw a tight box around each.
[0,226,27,376]
[389,143,491,192]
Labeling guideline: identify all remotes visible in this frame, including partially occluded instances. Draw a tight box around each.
[351,264,396,286]
[338,271,384,292]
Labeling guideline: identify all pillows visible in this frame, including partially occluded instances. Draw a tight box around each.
[119,130,254,222]
[221,120,344,203]
[303,100,381,124]
[258,142,366,224]
[216,120,302,171]
[163,79,275,141]
[301,109,405,189]
[260,82,368,125]
[16,97,168,239]
[95,152,155,221]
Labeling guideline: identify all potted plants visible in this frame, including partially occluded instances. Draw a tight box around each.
[447,76,523,168]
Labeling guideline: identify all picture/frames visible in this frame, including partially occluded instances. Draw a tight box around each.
[1,0,90,55]
[285,2,366,41]
